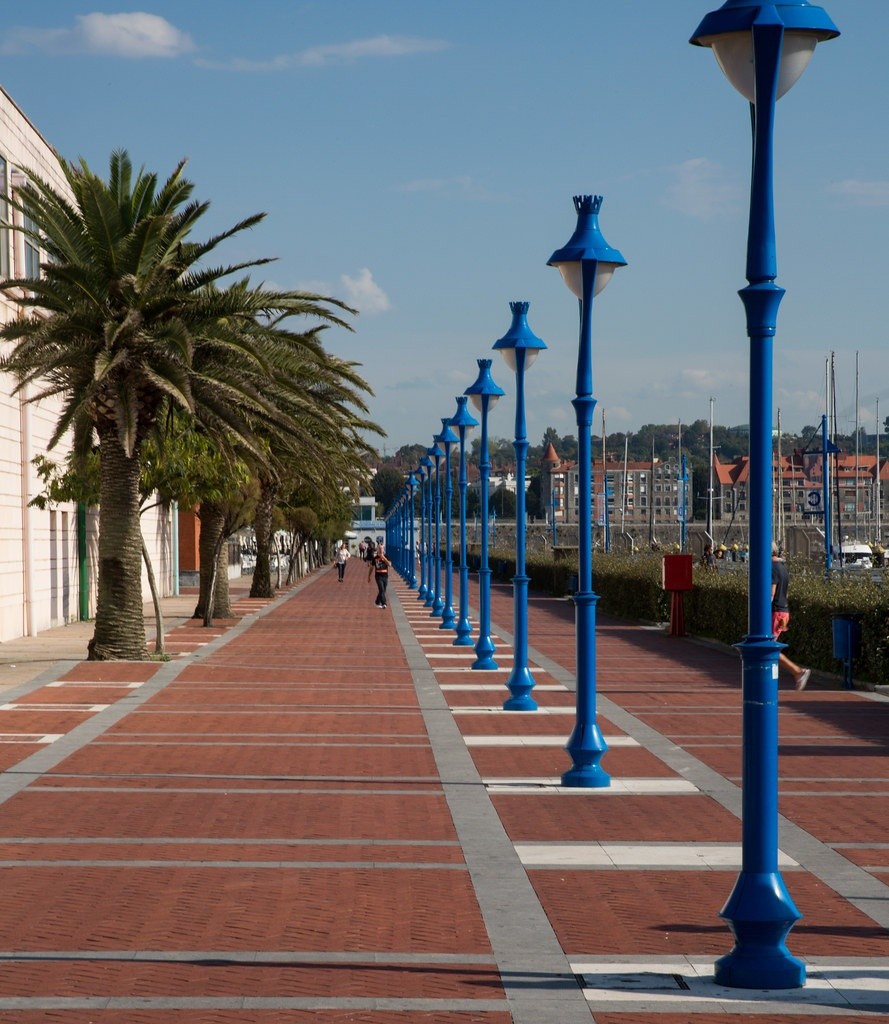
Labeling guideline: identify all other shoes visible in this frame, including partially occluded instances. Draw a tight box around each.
[338,579,344,583]
[383,604,387,609]
[375,604,383,609]
[795,668,812,693]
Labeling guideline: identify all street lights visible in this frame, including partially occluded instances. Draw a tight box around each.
[463,358,507,672]
[435,418,460,630]
[492,301,550,712]
[426,440,446,617]
[404,469,420,590]
[382,485,409,586]
[419,455,435,607]
[445,397,480,646]
[543,192,615,790]
[685,2,846,991]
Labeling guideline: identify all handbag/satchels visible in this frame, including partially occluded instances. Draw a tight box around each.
[334,564,338,569]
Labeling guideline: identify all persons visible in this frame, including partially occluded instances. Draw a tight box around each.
[367,545,391,609]
[432,543,435,555]
[415,541,419,562]
[367,542,376,566]
[704,544,713,566]
[771,542,811,690]
[359,540,367,560]
[335,543,351,582]
[715,549,722,558]
[727,548,737,562]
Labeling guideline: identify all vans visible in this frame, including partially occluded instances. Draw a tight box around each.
[829,542,874,573]
